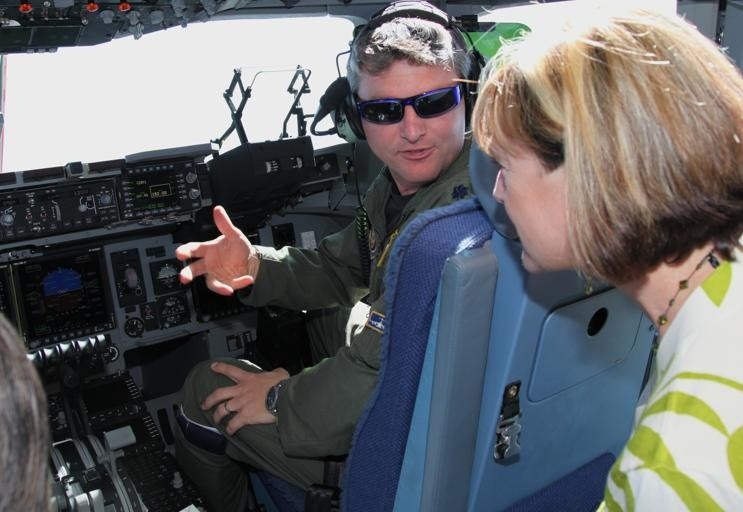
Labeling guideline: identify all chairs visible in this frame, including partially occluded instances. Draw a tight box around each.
[338,135,655,512]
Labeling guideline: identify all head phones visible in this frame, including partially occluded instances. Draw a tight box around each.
[331,8,486,144]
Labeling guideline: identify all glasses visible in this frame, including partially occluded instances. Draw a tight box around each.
[355,81,463,124]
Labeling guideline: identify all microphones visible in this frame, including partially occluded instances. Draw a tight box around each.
[309,76,351,135]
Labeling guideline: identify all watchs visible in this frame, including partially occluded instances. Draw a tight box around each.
[265,380,282,415]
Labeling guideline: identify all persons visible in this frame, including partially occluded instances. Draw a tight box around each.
[0,311,51,512]
[470,0,743,512]
[174,1,481,512]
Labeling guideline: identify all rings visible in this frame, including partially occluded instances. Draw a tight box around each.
[224,401,232,413]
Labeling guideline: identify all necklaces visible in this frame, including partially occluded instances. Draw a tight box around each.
[651,249,704,356]
